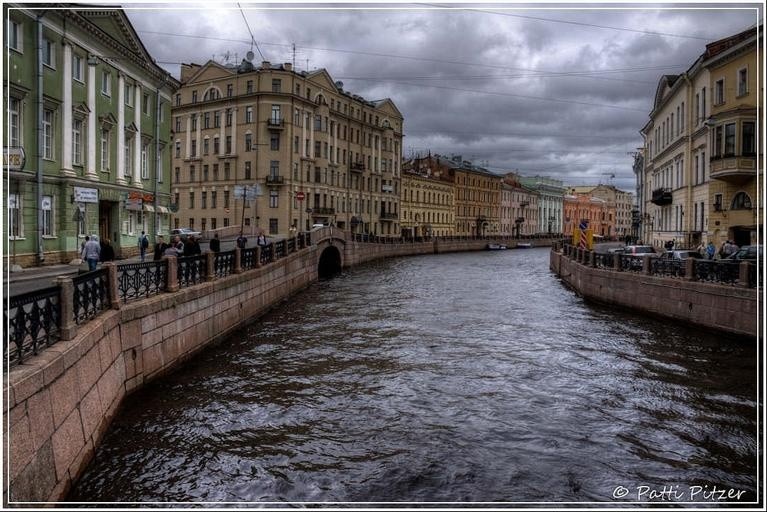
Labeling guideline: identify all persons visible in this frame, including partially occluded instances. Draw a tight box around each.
[717,238,739,259]
[136,231,148,263]
[696,240,706,260]
[183,236,201,282]
[80,234,102,272]
[236,232,247,248]
[171,235,184,283]
[98,238,115,264]
[209,233,220,251]
[163,243,178,257]
[153,234,168,262]
[666,238,675,250]
[256,231,266,246]
[705,241,715,260]
[624,234,643,247]
[288,224,296,238]
[80,235,89,263]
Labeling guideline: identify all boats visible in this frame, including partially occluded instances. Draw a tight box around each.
[517,243,532,248]
[487,241,508,251]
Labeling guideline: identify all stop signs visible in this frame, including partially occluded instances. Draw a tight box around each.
[296,191,306,202]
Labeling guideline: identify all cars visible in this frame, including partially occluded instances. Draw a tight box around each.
[606,244,763,286]
[170,228,203,243]
